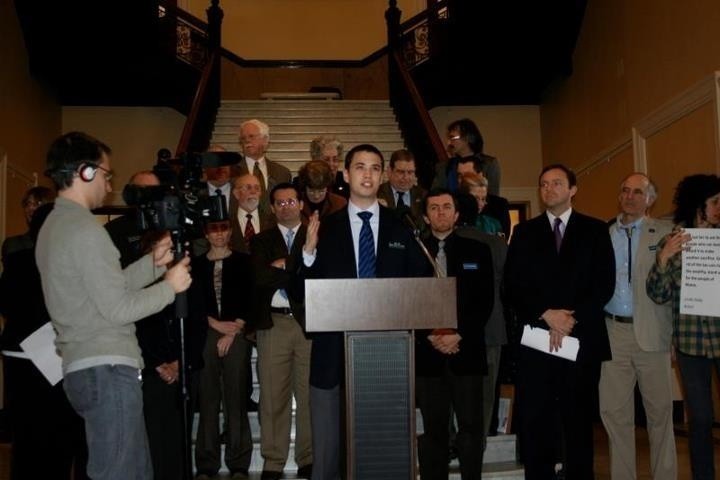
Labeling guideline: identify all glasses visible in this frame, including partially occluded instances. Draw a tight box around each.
[275,199,295,207]
[85,162,114,181]
[308,191,325,196]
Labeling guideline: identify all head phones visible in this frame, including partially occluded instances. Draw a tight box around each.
[44,162,95,182]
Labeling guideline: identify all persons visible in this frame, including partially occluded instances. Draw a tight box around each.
[35,132,193,480]
[101,117,510,480]
[2,187,75,480]
[501,163,615,480]
[645,173,719,480]
[599,172,679,479]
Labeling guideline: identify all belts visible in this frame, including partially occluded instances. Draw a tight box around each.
[603,310,633,323]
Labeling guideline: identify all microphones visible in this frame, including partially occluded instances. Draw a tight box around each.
[398,207,420,241]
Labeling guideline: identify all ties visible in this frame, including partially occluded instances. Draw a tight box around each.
[553,218,562,254]
[215,189,224,220]
[253,161,267,203]
[356,211,377,278]
[243,214,256,250]
[396,191,404,214]
[279,228,294,300]
[433,241,448,278]
